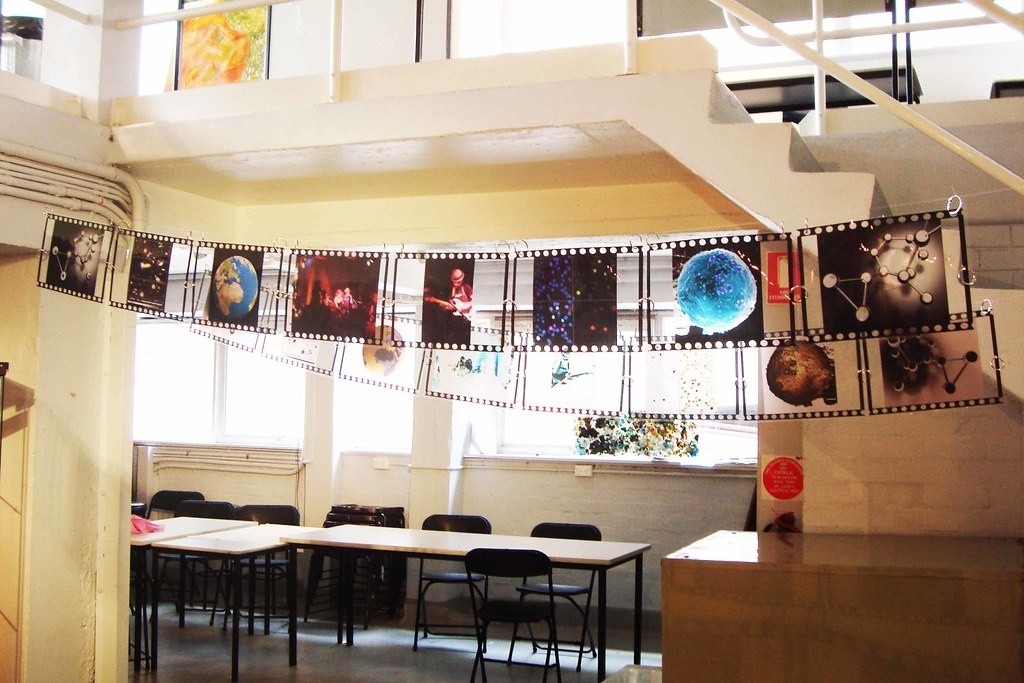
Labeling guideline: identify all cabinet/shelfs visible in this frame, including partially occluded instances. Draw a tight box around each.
[661,531,1024,683]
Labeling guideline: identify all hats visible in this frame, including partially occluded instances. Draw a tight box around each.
[451,269,465,282]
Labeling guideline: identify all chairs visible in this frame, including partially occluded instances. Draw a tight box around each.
[129,487,300,662]
[465,548,561,683]
[505,522,601,671]
[412,514,491,653]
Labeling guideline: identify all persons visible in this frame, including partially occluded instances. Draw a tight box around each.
[439,269,471,345]
[292,287,377,338]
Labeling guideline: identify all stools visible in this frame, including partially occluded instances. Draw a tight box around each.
[303,504,409,634]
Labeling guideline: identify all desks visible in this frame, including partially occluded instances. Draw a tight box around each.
[130,517,260,673]
[279,526,655,683]
[149,522,327,681]
[726,62,924,122]
